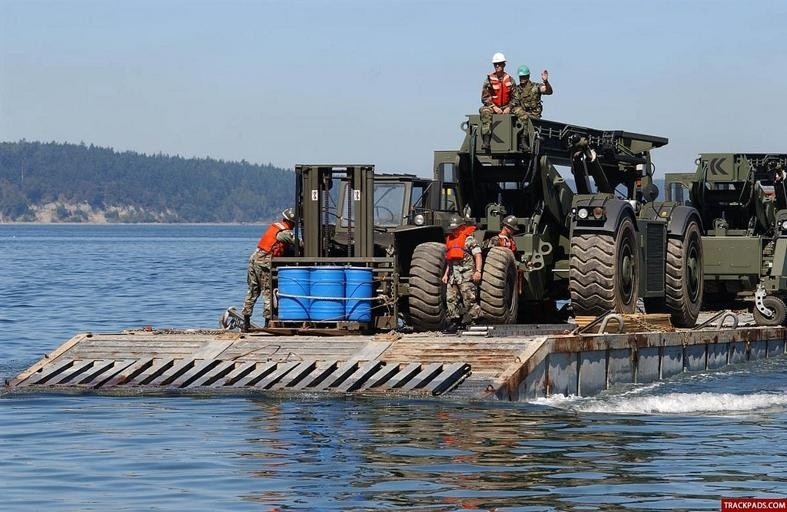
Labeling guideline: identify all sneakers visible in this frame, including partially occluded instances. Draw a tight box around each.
[443,321,459,333]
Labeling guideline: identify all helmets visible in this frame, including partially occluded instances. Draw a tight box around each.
[502,215,520,231]
[447,214,466,229]
[517,65,530,76]
[281,207,297,223]
[492,52,505,63]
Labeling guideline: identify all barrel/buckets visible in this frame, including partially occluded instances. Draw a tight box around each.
[346,265,373,322]
[276,266,308,320]
[308,265,345,320]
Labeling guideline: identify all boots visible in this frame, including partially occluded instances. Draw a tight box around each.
[480,133,491,150]
[517,134,532,153]
[242,316,251,330]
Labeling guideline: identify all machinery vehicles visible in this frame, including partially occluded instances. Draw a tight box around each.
[659,148,787,332]
[403,107,716,338]
[260,161,454,331]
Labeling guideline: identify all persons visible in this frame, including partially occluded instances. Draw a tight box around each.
[476,215,518,256]
[442,213,489,334]
[516,65,553,120]
[242,207,304,329]
[478,52,531,150]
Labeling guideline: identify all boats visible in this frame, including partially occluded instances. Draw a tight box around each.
[0,310,787,406]
